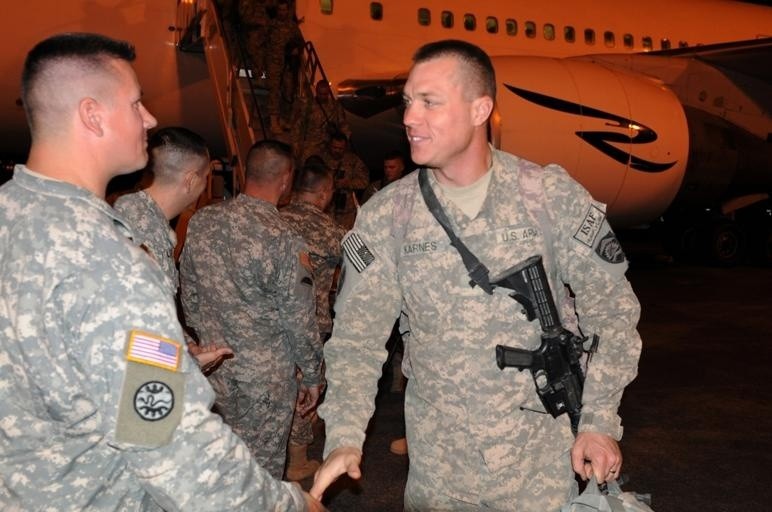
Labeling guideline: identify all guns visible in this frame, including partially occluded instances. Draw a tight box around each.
[488,254,608,496]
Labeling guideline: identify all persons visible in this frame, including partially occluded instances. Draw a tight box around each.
[0,30,324,512]
[104,126,234,375]
[179,1,409,483]
[310,37,644,510]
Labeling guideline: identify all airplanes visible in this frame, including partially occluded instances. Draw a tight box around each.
[1,0,771,259]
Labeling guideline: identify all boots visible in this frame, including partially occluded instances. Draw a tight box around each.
[286,444,321,481]
